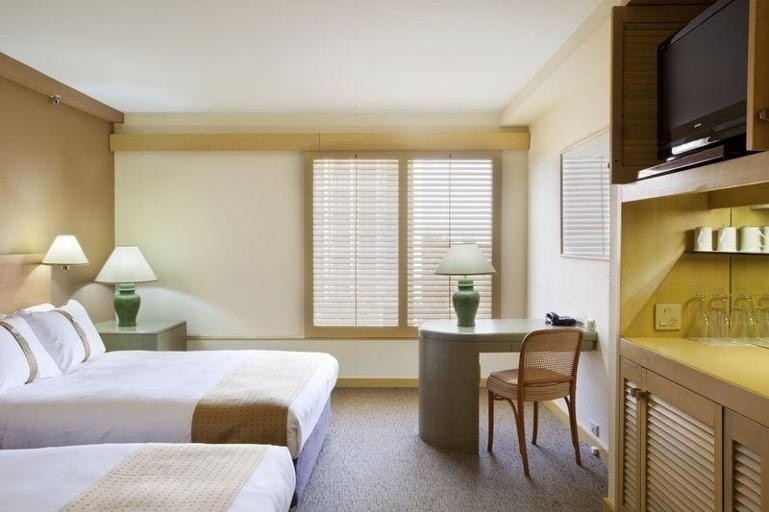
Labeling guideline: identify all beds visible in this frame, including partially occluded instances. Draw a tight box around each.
[0,254,339,507]
[0,443,295,512]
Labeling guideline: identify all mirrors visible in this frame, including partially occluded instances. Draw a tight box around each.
[559,129,609,261]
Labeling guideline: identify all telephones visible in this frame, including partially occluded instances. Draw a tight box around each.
[545,312,576,326]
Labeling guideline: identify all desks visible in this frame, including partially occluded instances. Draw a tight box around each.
[419,319,592,454]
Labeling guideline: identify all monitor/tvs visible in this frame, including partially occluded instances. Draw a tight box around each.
[656,0,748,159]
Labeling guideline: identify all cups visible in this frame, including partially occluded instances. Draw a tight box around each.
[692,226,738,254]
[739,226,768,253]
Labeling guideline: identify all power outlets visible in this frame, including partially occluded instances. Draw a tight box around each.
[656,304,682,331]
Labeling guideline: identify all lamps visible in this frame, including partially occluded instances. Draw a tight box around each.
[94,247,158,326]
[40,234,89,272]
[434,243,496,327]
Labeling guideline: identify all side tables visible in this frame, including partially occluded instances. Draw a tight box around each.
[96,320,186,351]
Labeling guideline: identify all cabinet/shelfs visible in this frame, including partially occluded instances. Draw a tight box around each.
[603,0,769,512]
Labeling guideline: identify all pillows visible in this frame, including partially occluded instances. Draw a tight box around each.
[0,298,107,388]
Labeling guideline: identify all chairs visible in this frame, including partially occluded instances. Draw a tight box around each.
[486,329,584,476]
